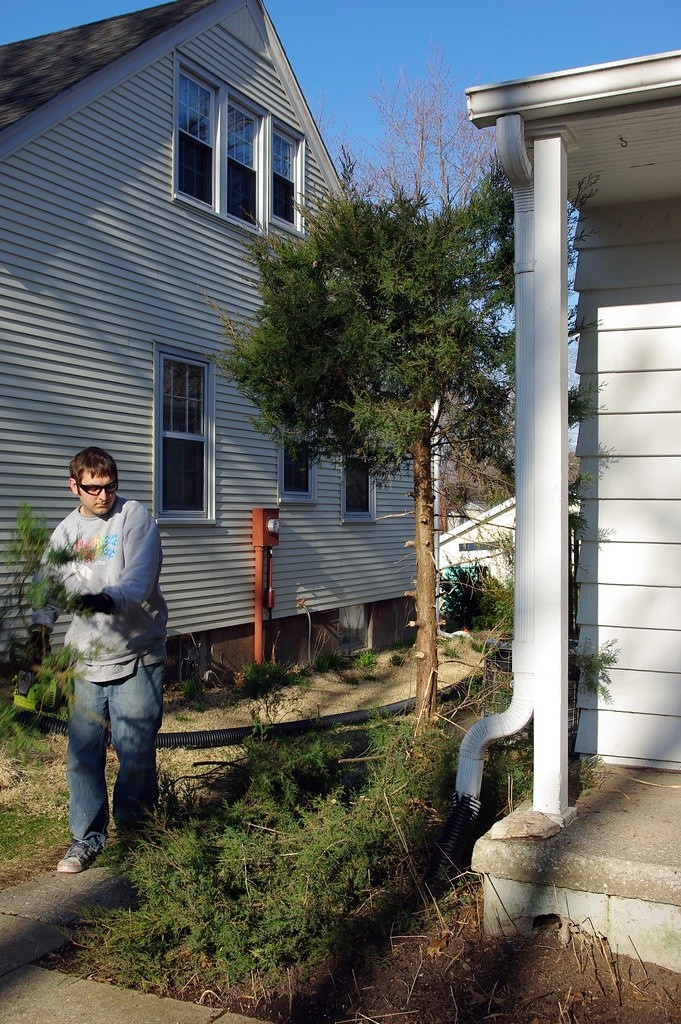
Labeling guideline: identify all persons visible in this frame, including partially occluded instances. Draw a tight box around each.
[21,446,167,874]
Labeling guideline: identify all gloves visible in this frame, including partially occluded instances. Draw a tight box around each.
[25,631,51,663]
[73,593,115,615]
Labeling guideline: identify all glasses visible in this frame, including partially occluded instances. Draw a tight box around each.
[77,481,118,497]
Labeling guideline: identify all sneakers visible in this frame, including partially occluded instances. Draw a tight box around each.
[56,842,94,874]
